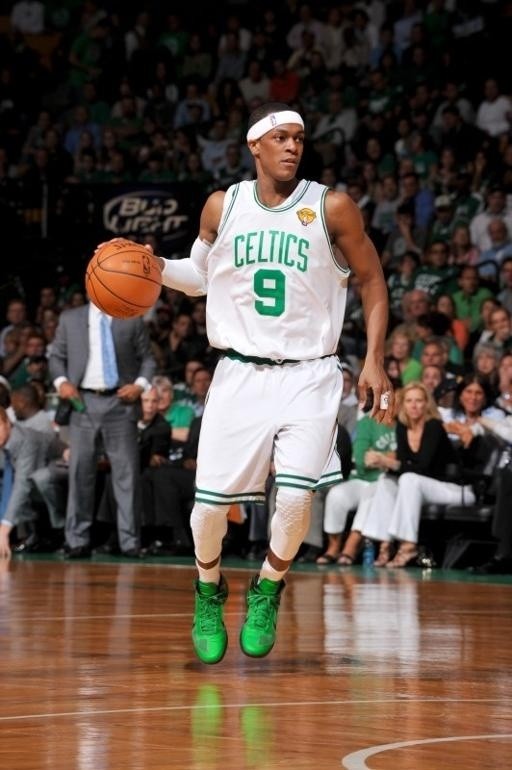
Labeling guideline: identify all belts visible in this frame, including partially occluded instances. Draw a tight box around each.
[76,384,123,399]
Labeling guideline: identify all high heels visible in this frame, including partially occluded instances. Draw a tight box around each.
[373,550,392,567]
[386,549,419,569]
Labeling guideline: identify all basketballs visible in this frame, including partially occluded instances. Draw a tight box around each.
[85,239,163,319]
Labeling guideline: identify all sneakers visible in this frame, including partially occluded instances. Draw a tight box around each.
[239,573,285,660]
[191,576,229,665]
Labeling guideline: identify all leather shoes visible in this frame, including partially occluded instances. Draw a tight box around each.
[121,548,146,560]
[65,545,92,560]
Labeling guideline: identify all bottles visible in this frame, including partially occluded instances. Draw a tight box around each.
[362,542,376,568]
[70,396,87,413]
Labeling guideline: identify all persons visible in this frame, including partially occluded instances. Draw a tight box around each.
[0,0,512,575]
[95,102,396,664]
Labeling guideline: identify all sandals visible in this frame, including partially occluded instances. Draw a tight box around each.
[316,553,335,565]
[337,554,355,566]
